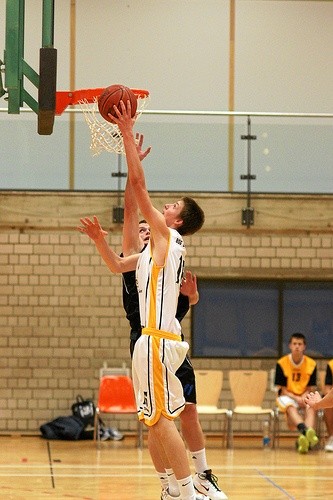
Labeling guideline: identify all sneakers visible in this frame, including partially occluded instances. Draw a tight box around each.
[162,481,210,500]
[193,469,228,500]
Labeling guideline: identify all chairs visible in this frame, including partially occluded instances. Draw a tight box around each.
[194,372,333,452]
[92,375,141,450]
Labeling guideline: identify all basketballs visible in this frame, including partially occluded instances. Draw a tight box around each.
[98,84,137,124]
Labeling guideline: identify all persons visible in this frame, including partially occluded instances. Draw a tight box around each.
[273,333,318,454]
[119,133,228,500]
[303,391,333,410]
[324,359,333,452]
[77,100,204,500]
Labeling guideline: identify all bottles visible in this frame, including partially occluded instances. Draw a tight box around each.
[263,421,270,450]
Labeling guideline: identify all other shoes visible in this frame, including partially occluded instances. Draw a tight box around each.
[298,436,309,454]
[109,431,125,441]
[326,442,333,451]
[305,429,319,448]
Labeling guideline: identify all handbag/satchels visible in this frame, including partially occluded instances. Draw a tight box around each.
[40,399,102,439]
